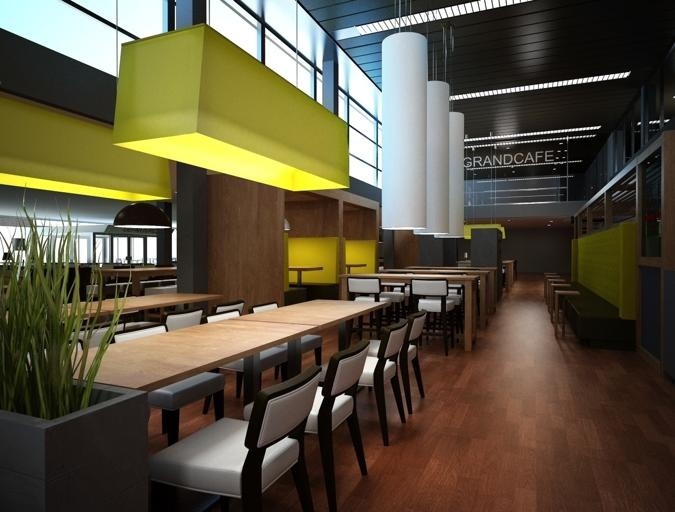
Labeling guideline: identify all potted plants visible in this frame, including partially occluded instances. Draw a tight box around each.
[2,182,150,512]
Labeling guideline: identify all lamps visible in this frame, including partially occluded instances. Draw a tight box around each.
[381,0,465,239]
[112,198,171,231]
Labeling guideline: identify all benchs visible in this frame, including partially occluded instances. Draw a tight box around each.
[551,277,636,352]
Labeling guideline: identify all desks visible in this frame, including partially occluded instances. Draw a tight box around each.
[337,263,498,353]
